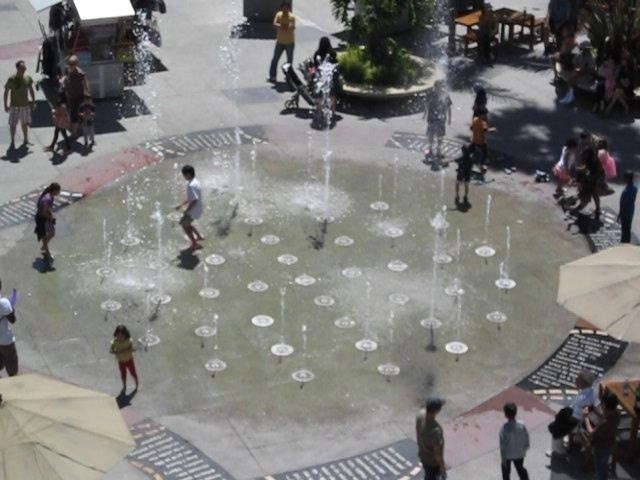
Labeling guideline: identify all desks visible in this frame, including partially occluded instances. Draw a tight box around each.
[454,10,482,57]
[606,380,640,463]
[495,8,534,53]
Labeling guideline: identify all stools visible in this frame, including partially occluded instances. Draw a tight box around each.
[523,20,544,41]
[463,31,498,60]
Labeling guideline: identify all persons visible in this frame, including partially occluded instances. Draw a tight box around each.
[33,180,63,260]
[264,2,297,87]
[412,393,449,480]
[58,53,90,140]
[616,171,638,243]
[470,81,489,119]
[466,104,498,175]
[3,59,36,152]
[108,321,141,389]
[308,35,343,127]
[544,369,597,459]
[453,140,475,205]
[76,93,97,147]
[497,400,531,480]
[421,78,453,162]
[580,390,622,480]
[0,278,19,377]
[173,162,205,252]
[529,0,640,215]
[42,91,73,155]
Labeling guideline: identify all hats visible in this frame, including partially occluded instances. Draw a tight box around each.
[427,396,445,409]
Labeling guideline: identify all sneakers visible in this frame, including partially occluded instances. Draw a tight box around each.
[545,450,554,457]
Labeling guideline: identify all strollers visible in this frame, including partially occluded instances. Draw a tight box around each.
[279,58,332,120]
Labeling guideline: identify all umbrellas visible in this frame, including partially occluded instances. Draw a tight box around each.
[553,241,640,343]
[0,371,136,479]
